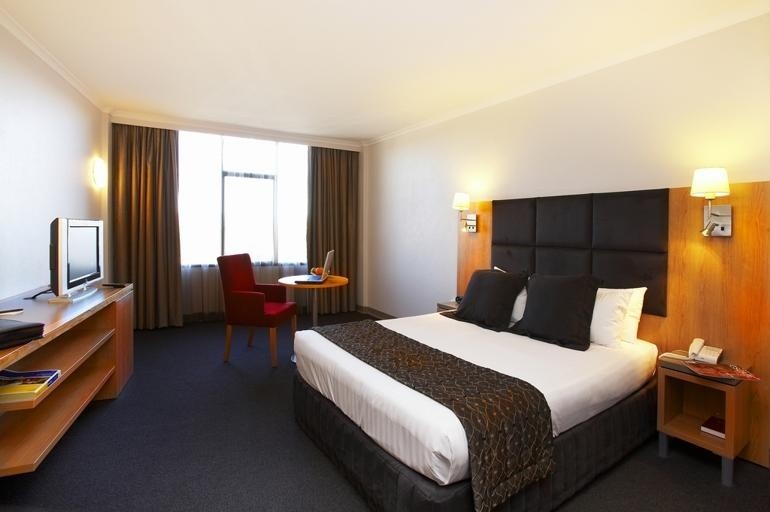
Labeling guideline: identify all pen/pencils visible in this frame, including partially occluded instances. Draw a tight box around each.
[0,309,23,313]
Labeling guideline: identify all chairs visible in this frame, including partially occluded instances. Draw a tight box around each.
[216,252,300,369]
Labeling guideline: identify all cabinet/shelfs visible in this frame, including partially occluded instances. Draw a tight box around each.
[1,281,136,479]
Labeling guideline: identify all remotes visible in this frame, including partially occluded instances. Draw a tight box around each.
[102,283,126,288]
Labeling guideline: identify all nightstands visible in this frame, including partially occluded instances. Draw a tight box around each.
[656,348,753,488]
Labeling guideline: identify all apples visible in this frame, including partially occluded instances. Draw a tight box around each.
[315,267,323,275]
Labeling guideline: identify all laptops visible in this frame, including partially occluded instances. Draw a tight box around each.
[295,250,335,284]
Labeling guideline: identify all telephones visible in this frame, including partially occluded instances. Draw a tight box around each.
[688,338,723,365]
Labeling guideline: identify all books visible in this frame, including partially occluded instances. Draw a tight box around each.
[700,415,725,440]
[0,368,62,404]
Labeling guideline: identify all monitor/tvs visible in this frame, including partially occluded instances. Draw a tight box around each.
[48,218,104,303]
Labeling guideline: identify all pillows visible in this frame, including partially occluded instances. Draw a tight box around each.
[453,265,648,352]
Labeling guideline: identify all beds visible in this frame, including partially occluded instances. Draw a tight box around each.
[293,309,658,511]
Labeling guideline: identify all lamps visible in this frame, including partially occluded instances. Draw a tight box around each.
[451,192,477,233]
[689,167,733,239]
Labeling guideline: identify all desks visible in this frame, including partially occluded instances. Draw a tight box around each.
[436,300,460,312]
[277,273,350,364]
